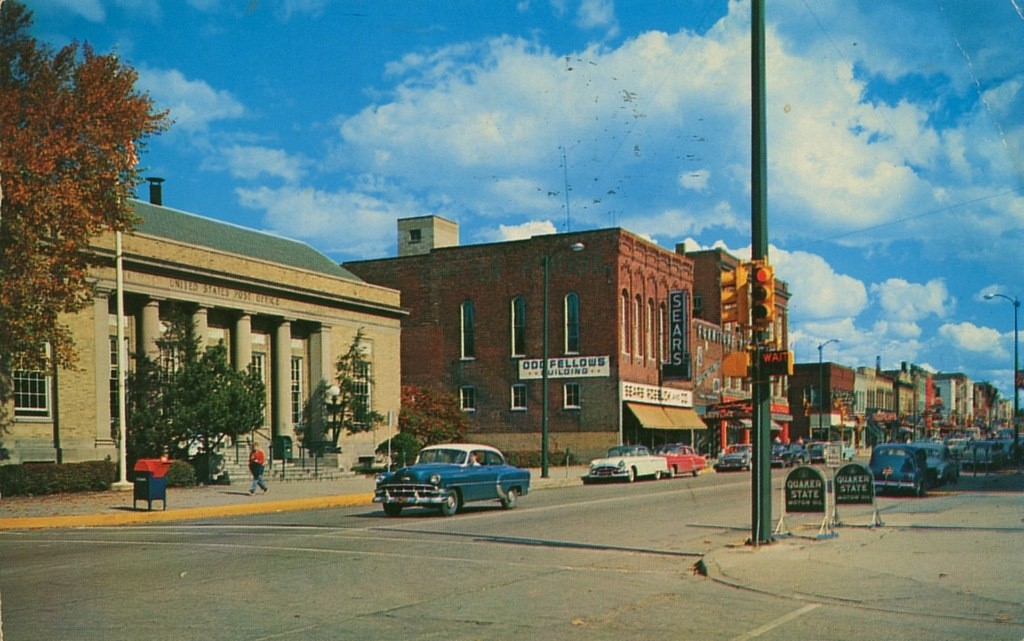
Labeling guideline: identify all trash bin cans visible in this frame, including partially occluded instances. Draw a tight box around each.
[132,459,173,510]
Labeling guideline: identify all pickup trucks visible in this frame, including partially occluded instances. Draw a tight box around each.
[588,446,668,483]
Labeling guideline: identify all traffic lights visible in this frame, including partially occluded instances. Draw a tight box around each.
[753,265,774,323]
[761,350,794,376]
[720,266,749,324]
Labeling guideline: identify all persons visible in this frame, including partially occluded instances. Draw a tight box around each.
[249,443,268,494]
[470,452,480,467]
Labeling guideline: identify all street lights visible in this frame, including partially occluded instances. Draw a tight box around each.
[329,386,340,454]
[817,338,842,442]
[540,243,585,478]
[984,292,1019,469]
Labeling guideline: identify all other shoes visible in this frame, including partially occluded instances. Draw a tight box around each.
[263,488,269,494]
[248,490,255,496]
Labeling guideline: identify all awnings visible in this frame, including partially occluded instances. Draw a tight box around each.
[742,420,782,430]
[626,403,708,430]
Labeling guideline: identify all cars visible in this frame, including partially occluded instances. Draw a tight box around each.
[372,443,531,516]
[915,442,957,488]
[808,442,830,463]
[787,443,811,467]
[652,444,708,479]
[718,444,753,471]
[867,444,928,498]
[961,435,1024,469]
[769,444,795,468]
